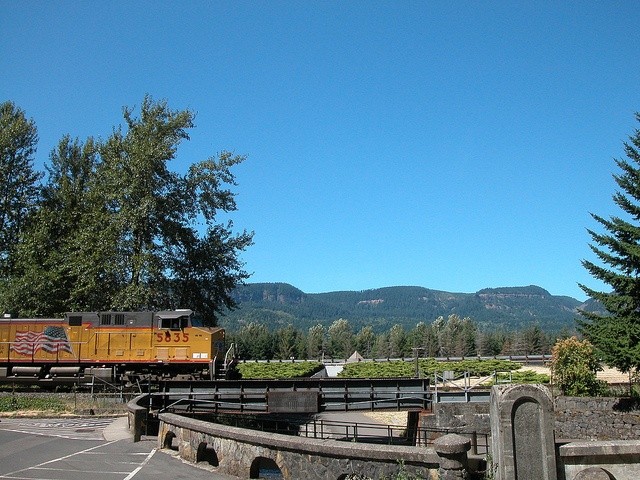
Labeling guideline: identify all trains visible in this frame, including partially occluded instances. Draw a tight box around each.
[0,309,242,386]
